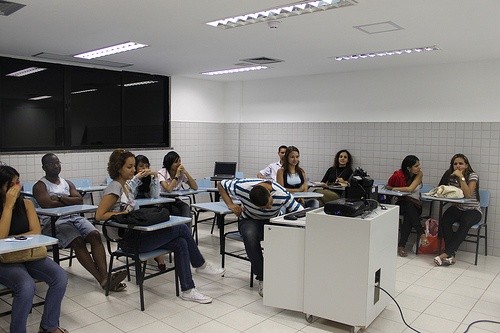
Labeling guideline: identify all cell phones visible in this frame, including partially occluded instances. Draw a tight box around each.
[15,236,26,239]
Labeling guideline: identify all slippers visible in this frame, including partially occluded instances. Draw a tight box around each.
[102,282,127,292]
[39,327,69,333]
[104,270,128,288]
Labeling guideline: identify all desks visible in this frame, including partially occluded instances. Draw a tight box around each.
[75,186,108,192]
[160,189,206,196]
[190,199,244,215]
[371,186,413,196]
[20,191,34,198]
[0,232,60,255]
[34,205,98,217]
[99,215,192,231]
[290,191,324,198]
[133,196,176,206]
[206,187,220,193]
[421,194,470,203]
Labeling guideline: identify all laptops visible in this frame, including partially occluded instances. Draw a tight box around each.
[211,162,236,179]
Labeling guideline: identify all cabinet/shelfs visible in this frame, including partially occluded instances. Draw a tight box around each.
[262,204,400,333]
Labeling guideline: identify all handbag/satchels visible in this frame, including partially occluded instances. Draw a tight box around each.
[348,166,374,187]
[424,185,464,199]
[412,218,446,254]
[0,246,47,263]
[110,208,169,227]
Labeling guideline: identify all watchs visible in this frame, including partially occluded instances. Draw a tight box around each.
[56,194,63,201]
[460,177,465,181]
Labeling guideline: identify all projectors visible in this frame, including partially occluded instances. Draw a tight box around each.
[324,199,377,217]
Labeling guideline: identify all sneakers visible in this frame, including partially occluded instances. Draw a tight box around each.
[182,287,213,303]
[194,261,225,275]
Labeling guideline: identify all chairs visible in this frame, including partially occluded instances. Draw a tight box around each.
[0,172,490,318]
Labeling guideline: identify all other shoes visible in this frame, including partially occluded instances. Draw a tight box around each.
[258,280,263,297]
[399,246,407,257]
[434,256,442,266]
[442,256,455,265]
[154,257,166,271]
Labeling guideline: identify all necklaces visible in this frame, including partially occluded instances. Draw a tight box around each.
[335,166,348,183]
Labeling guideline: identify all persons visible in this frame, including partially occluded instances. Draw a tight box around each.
[312,149,354,209]
[385,154,428,257]
[257,145,289,188]
[94,149,226,304]
[156,151,198,239]
[0,165,70,333]
[127,155,161,203]
[31,151,131,293]
[276,146,307,208]
[430,153,482,266]
[215,174,305,299]
